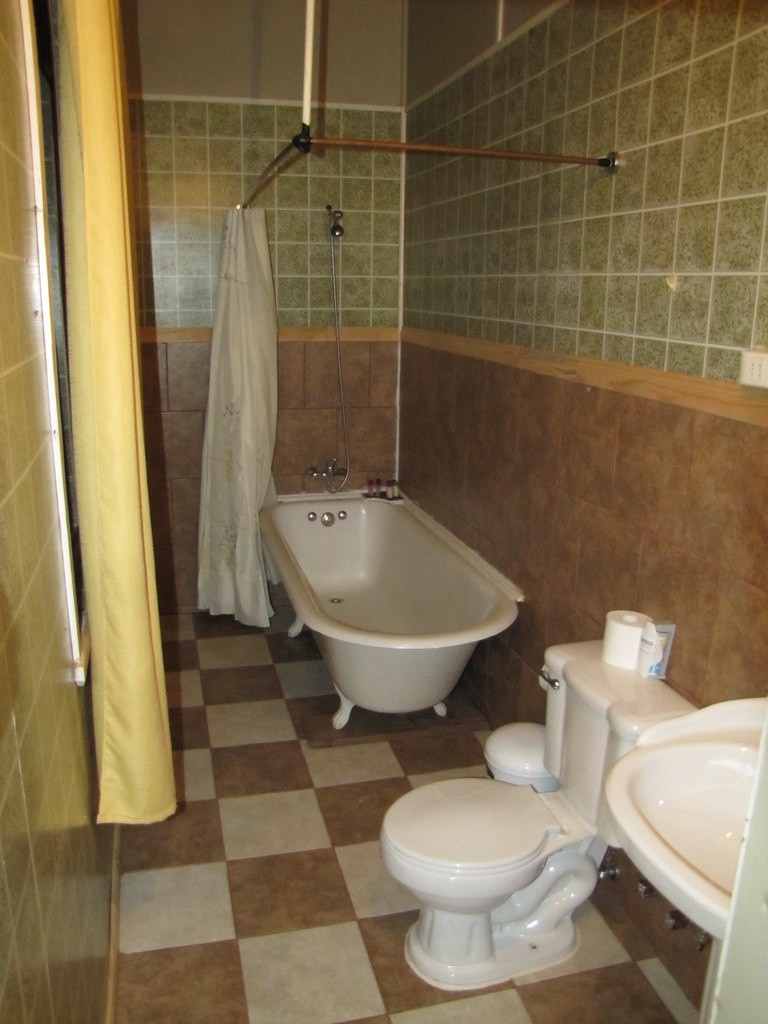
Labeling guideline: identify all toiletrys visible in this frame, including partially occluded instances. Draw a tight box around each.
[367,481,374,498]
[375,478,382,497]
[393,481,399,498]
[385,481,394,500]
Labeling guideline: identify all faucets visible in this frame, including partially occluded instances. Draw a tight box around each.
[324,456,339,494]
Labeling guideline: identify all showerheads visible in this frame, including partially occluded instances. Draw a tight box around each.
[329,221,346,238]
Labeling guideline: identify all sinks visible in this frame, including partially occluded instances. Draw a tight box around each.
[602,693,768,945]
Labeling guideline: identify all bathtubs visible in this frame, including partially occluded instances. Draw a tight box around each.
[260,480,525,733]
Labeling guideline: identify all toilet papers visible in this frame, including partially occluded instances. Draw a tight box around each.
[598,604,667,684]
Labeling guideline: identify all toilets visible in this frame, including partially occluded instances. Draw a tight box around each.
[377,638,699,996]
